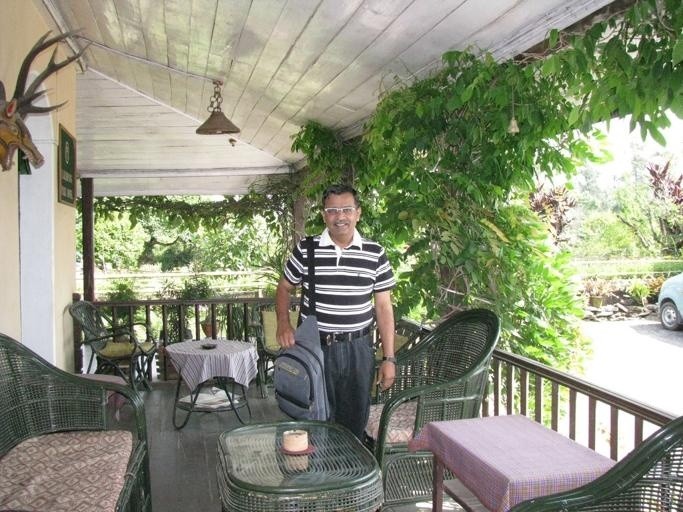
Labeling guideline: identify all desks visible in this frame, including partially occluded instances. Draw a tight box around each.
[407,414,643,512]
[164,337,257,430]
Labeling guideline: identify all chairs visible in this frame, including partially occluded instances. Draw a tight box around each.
[69,301,157,395]
[371,320,422,404]
[509,416,683,512]
[376,308,502,507]
[248,303,294,398]
[0,332,152,510]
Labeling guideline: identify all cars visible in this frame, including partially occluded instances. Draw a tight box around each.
[658,273,683,331]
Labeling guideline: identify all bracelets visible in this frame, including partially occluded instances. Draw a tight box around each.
[383,357,399,364]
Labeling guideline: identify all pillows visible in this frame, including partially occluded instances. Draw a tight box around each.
[259,308,298,353]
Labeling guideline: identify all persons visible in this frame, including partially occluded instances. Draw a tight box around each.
[273,183,398,456]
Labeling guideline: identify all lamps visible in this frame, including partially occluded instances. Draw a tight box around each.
[193,82,240,135]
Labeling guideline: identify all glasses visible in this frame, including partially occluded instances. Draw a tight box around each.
[324,206,360,216]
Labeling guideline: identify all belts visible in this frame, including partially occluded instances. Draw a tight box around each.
[319,323,372,343]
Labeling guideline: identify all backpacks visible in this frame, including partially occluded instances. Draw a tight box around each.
[273,314,331,422]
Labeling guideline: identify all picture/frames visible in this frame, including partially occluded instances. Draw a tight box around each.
[55,125,78,209]
